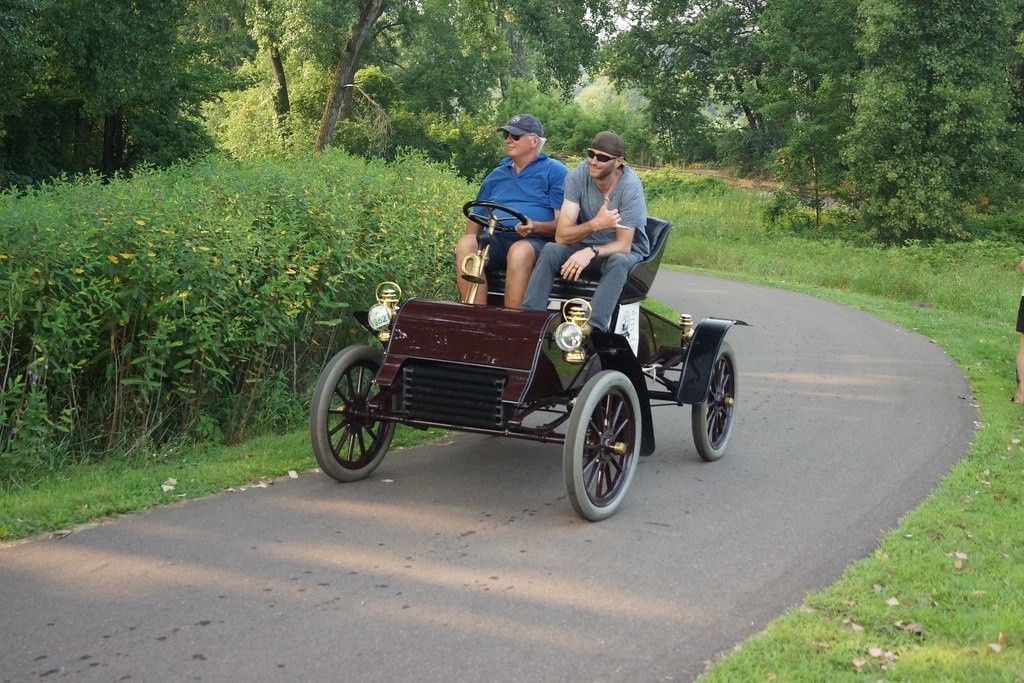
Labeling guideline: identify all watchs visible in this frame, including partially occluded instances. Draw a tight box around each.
[589,244,600,260]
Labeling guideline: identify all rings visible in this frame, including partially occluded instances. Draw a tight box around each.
[573,266,578,270]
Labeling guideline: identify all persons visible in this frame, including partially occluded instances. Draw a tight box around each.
[521,130,651,332]
[455,113,571,309]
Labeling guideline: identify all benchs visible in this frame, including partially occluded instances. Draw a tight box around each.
[485,216,673,305]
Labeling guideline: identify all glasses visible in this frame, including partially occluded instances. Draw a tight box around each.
[587,148,618,162]
[502,130,521,141]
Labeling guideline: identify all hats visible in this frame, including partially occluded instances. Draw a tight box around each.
[496,113,544,137]
[587,130,625,156]
